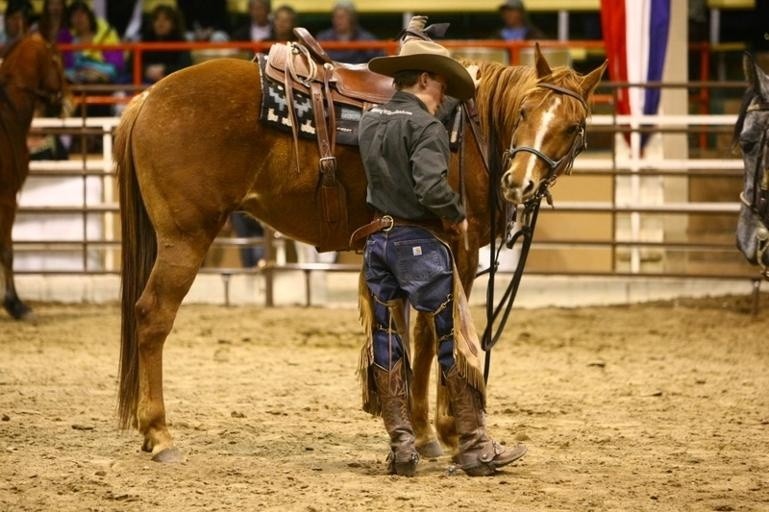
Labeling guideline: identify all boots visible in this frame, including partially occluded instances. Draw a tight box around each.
[372,356,419,477]
[440,364,527,477]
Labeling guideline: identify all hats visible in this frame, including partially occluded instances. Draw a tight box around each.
[368,38,476,102]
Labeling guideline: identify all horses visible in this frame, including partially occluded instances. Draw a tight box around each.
[110,38,612,464]
[0,22,68,327]
[729,50,769,275]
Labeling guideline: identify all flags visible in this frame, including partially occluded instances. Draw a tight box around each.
[600,0,674,158]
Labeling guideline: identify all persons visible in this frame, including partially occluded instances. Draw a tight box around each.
[1,1,384,267]
[495,0,543,40]
[346,29,530,479]
